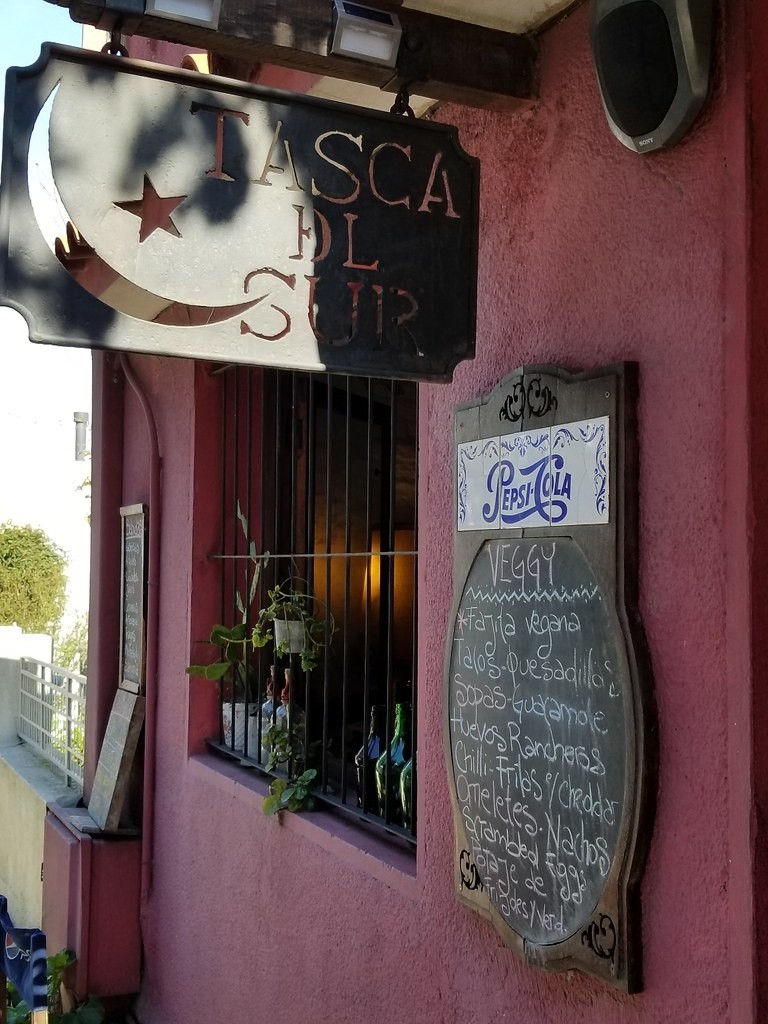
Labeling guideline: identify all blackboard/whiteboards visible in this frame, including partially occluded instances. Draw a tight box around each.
[116,502,149,697]
[439,354,663,997]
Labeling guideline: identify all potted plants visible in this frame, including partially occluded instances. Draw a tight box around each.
[182,575,340,767]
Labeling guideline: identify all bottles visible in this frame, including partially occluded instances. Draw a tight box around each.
[354,704,387,817]
[375,702,410,823]
[276,668,307,775]
[262,664,281,769]
[399,756,413,828]
